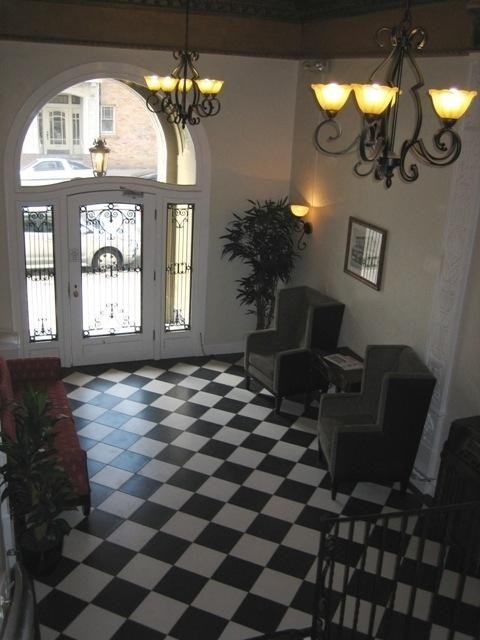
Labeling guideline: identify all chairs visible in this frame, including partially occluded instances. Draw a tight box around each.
[316,344,436,500]
[244,285,344,416]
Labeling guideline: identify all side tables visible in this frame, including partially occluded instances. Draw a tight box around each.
[317,362,364,393]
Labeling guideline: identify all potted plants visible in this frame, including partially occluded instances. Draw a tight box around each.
[0,383,79,576]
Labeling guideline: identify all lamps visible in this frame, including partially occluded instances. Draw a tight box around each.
[143,0,225,130]
[310,1,477,188]
[89,138,111,177]
[290,205,313,250]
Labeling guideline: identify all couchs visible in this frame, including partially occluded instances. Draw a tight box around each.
[0,355,90,514]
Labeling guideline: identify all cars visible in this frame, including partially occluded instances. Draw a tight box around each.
[20,156,158,274]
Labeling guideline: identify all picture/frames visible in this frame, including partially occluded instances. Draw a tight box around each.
[343,216,387,290]
